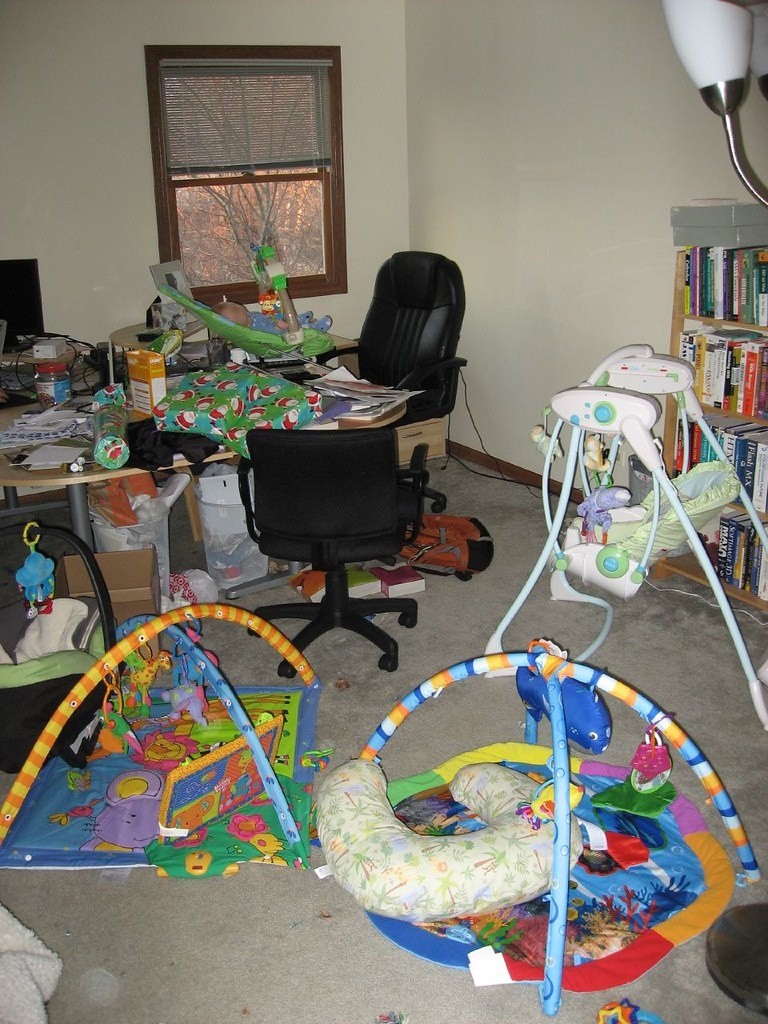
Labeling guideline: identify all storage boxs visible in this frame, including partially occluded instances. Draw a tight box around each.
[126,349,308,459]
[51,546,161,627]
[670,205,768,249]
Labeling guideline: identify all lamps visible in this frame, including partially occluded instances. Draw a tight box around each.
[662,0,768,210]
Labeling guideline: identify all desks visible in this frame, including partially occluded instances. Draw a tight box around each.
[0,327,407,555]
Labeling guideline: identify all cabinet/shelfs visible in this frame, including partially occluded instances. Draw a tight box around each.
[652,250,768,611]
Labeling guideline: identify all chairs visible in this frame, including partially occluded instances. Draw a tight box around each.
[236,427,446,679]
[309,251,467,427]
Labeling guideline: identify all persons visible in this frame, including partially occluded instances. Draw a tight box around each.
[210,301,333,335]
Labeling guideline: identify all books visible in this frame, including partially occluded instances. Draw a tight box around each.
[678,329,768,419]
[291,564,427,603]
[675,412,768,602]
[679,245,768,329]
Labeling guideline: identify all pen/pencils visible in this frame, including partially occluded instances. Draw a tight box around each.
[63,463,69,475]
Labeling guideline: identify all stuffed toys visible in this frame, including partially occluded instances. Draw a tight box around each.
[576,434,632,544]
[259,292,282,319]
[528,406,564,463]
[15,522,56,620]
[97,614,219,756]
[516,639,613,831]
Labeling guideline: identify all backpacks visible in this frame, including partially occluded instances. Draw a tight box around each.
[399,513,495,580]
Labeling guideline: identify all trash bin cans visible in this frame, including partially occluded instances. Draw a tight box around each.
[92,496,172,599]
[193,483,269,591]
